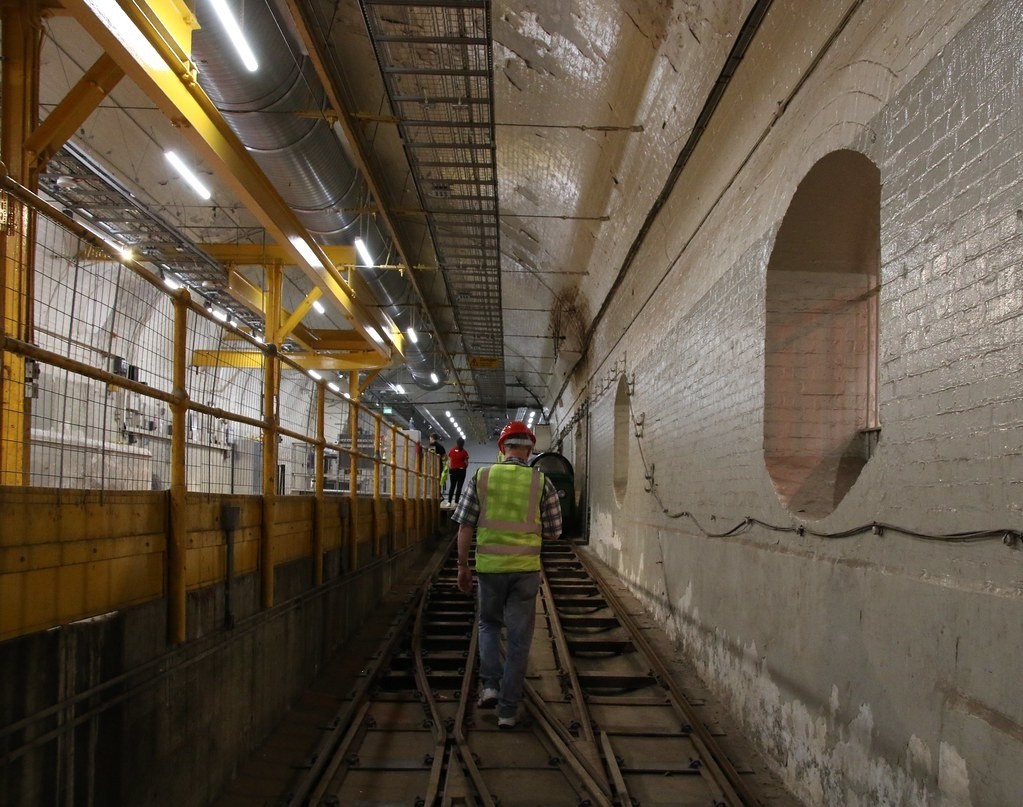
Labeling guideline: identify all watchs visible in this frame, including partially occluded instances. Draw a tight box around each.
[455,561,469,565]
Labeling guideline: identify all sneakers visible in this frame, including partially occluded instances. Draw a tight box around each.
[498,713,523,728]
[476,687,500,709]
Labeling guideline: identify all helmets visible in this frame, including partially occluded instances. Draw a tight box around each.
[497,421,536,455]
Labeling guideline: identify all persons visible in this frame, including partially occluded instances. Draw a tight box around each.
[447,435,468,506]
[428,434,448,505]
[449,422,563,730]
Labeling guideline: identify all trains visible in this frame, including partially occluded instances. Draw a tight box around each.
[528,451,575,517]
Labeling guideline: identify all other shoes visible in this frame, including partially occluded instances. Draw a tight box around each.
[446,502,451,507]
[454,502,458,507]
